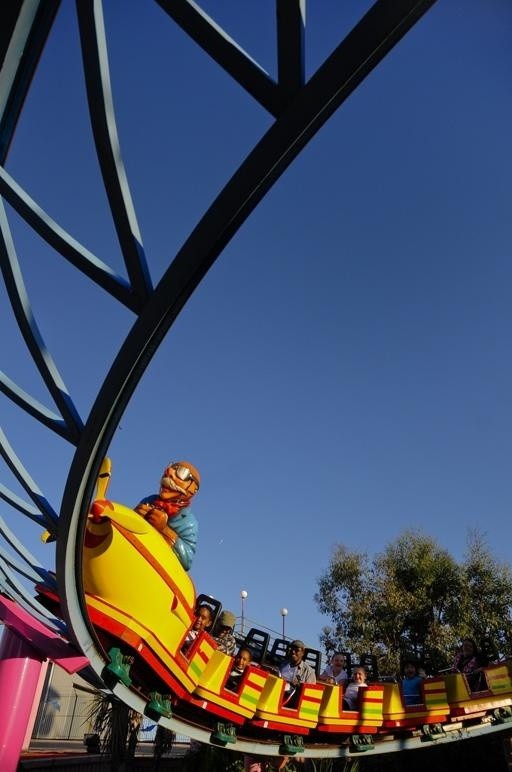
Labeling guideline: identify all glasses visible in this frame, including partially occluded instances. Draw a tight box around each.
[294,648,305,652]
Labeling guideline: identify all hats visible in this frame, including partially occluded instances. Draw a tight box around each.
[218,610,236,629]
[289,640,305,648]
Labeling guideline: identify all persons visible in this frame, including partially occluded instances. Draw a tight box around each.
[272,639,318,707]
[209,610,236,655]
[225,648,254,689]
[319,653,350,691]
[449,636,489,691]
[181,604,213,654]
[340,666,369,711]
[397,660,426,705]
[135,459,202,571]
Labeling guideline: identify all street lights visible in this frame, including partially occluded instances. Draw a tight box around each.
[241,591,247,633]
[281,608,288,656]
